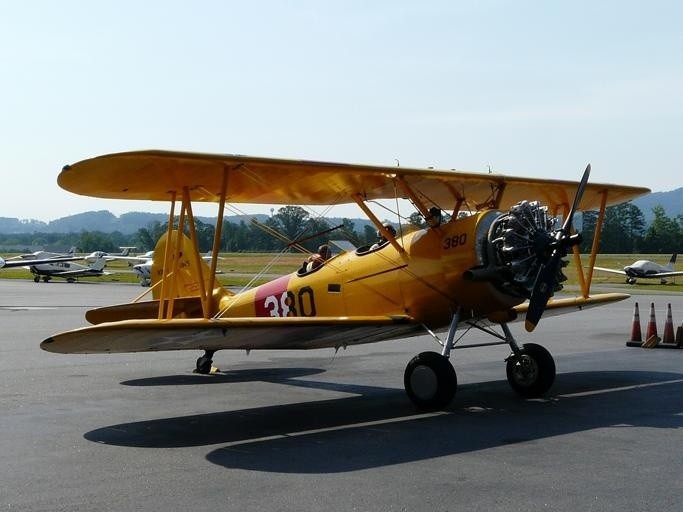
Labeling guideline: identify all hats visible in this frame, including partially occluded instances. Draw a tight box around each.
[428,207,441,213]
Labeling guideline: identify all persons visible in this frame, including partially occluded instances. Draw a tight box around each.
[304,244,331,273]
[367,222,397,250]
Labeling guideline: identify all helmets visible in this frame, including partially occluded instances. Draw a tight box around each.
[376,223,396,238]
[317,244,331,255]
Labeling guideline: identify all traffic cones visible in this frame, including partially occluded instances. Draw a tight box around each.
[623,299,679,347]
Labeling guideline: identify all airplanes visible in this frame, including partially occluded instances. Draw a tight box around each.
[585,250,683,287]
[41,148,652,412]
[0,243,229,289]
